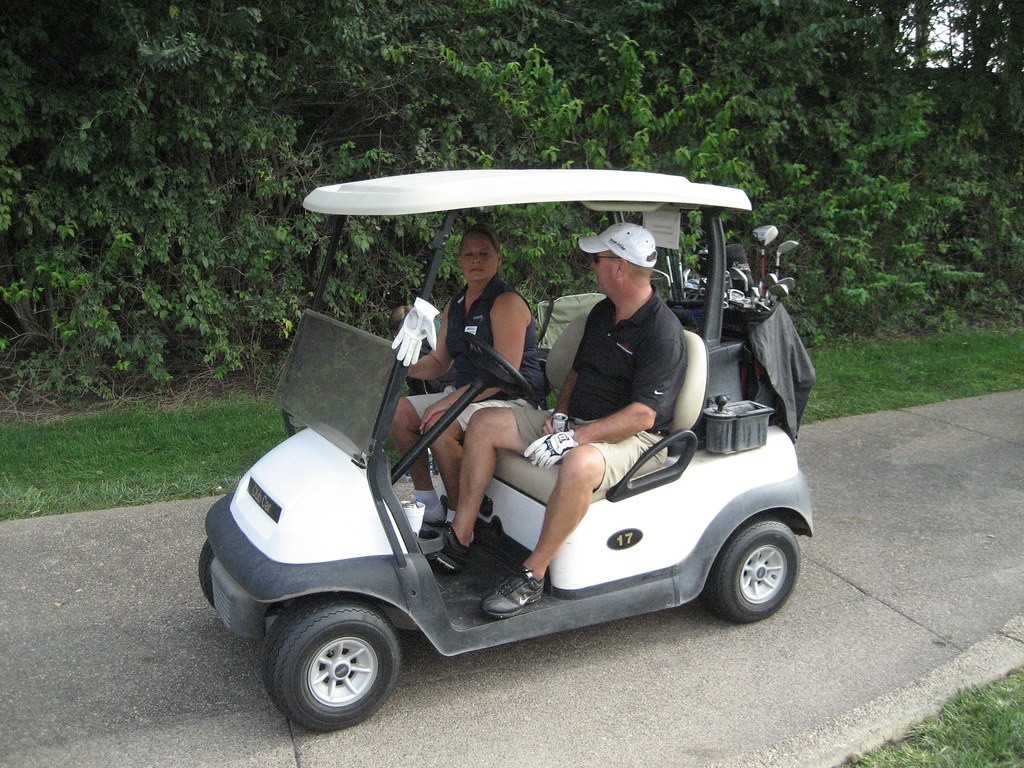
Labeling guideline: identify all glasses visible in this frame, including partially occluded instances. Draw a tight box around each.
[589,253,620,265]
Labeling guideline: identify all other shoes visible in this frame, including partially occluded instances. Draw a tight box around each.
[423,503,447,523]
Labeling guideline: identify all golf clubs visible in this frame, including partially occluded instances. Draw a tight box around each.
[678,221,799,316]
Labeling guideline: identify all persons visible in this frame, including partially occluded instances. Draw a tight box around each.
[386,222,688,618]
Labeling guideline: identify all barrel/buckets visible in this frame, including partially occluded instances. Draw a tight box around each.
[400,500,425,538]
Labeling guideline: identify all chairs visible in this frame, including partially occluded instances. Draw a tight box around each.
[492,315,708,510]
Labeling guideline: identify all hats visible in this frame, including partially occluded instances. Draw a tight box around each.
[579,223,658,268]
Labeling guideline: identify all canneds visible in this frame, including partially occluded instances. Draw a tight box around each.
[552,412,568,434]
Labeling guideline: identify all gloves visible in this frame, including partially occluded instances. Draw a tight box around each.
[551,411,571,434]
[523,428,579,469]
[391,296,440,366]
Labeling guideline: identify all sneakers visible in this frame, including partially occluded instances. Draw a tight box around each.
[479,564,544,619]
[426,526,471,572]
